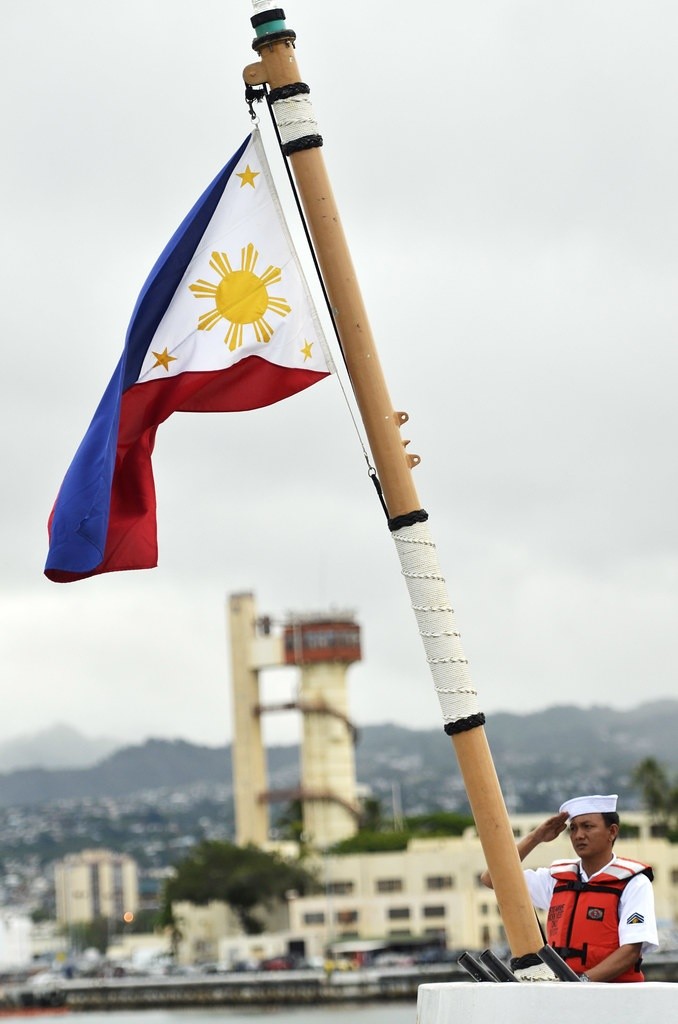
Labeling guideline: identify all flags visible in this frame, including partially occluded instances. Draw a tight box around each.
[44,129,333,582]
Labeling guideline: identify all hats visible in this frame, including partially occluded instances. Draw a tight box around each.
[559,795,618,821]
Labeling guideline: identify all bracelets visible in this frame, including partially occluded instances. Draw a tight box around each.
[577,973,590,982]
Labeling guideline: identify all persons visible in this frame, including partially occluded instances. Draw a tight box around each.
[481,795,660,983]
